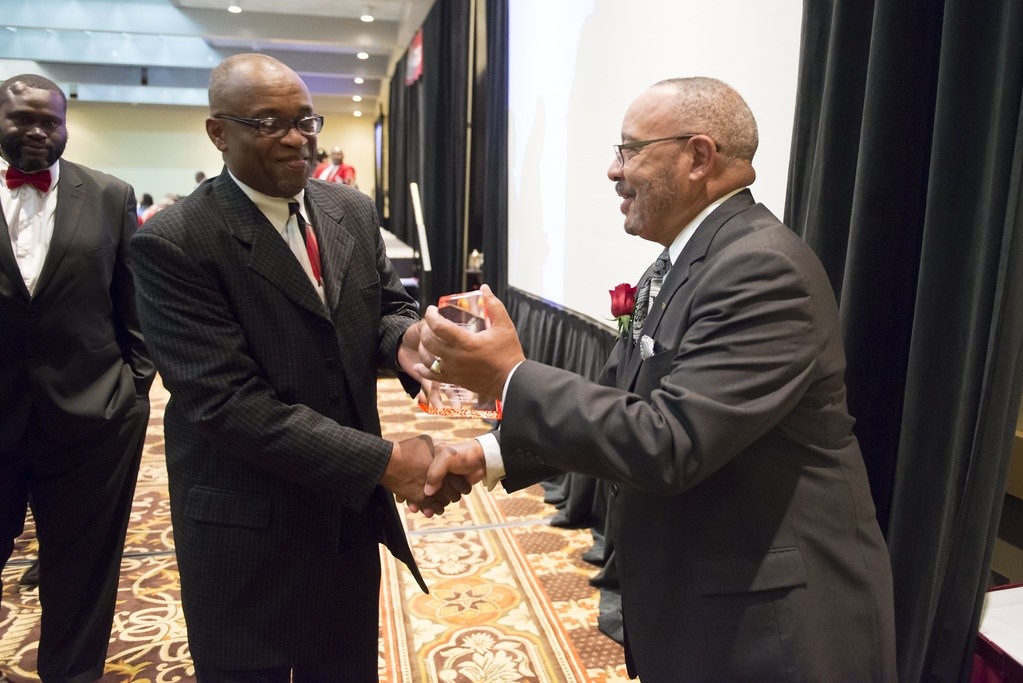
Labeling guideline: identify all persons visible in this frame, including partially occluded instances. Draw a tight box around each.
[135,194,154,217]
[130,52,473,683]
[310,147,355,187]
[392,74,896,683]
[0,74,156,683]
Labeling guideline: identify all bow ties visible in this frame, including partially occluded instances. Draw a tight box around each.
[6,166,51,193]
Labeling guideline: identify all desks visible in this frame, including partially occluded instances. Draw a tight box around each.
[969,583,1023,683]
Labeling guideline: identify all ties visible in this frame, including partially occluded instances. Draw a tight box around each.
[289,203,325,297]
[633,247,672,350]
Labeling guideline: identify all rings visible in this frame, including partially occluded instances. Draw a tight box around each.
[430,360,441,374]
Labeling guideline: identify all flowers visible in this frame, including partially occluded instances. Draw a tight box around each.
[606,282,639,342]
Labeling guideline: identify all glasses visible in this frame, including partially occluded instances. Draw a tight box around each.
[215,114,324,138]
[614,134,721,167]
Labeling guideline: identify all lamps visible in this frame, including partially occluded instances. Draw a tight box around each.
[360,6,374,22]
[227,0,242,13]
